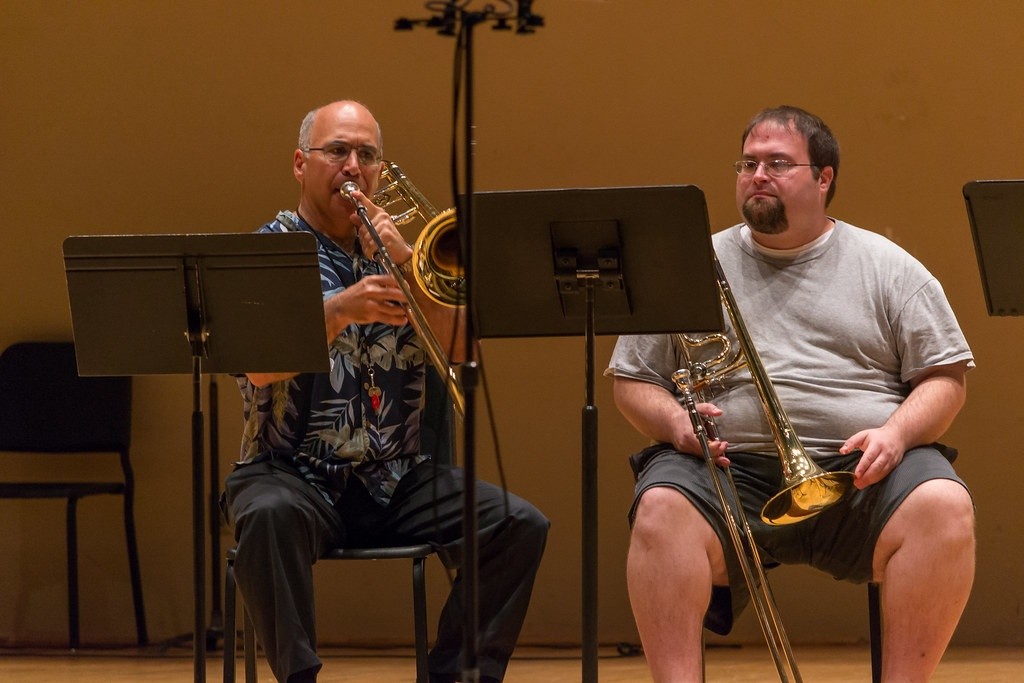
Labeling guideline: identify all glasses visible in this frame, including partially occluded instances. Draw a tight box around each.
[308,142,382,164]
[734,160,817,176]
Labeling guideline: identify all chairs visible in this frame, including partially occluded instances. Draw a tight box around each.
[222,361,481,683]
[0,341,150,650]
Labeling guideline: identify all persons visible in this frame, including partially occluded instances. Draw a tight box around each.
[605,106,977,683]
[218,100,551,683]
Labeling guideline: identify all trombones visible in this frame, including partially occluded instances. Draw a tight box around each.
[341,160,468,416]
[669,246,855,683]
